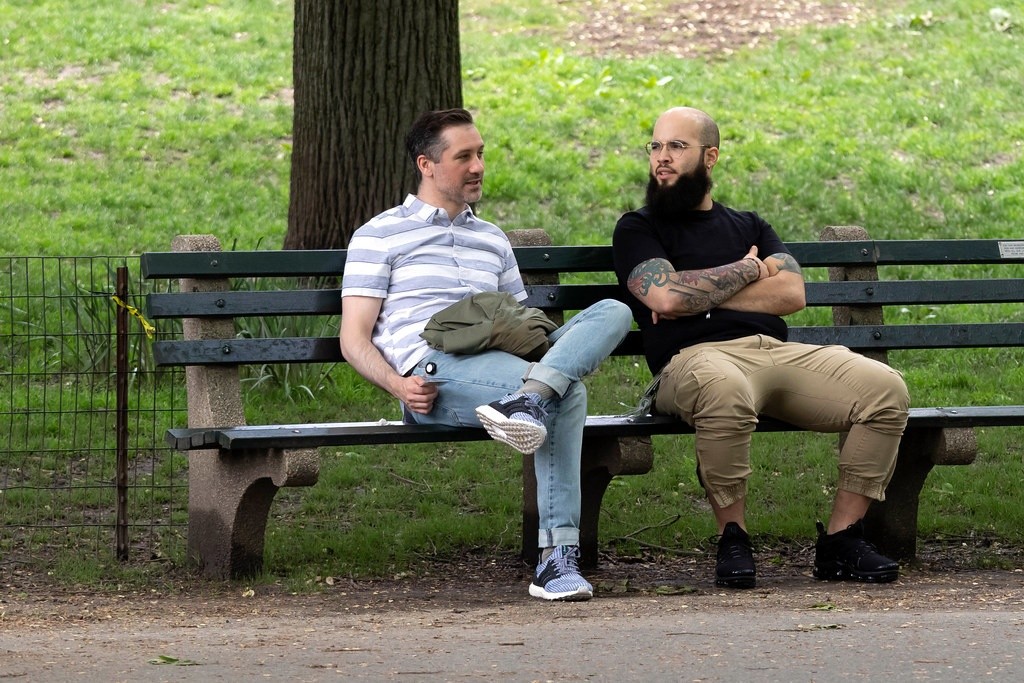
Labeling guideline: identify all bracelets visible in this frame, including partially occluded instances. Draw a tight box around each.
[744,257,761,281]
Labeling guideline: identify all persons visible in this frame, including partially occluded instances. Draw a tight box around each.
[613,107,911,589]
[341,109,633,601]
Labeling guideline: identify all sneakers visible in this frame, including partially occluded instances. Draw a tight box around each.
[708,521,757,588]
[475,392,548,454]
[813,518,898,582]
[528,545,593,601]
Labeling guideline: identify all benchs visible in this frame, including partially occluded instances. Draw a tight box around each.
[141,227,1024,584]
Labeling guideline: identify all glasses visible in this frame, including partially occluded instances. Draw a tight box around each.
[645,140,711,158]
